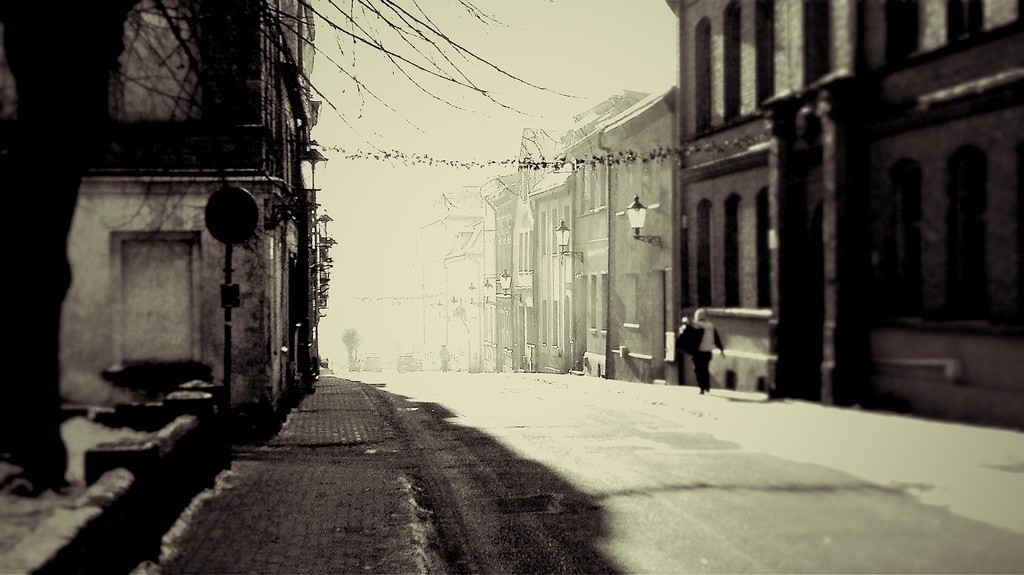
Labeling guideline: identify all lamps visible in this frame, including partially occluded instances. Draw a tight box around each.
[483,278,493,301]
[496,268,512,293]
[627,196,648,236]
[468,282,477,303]
[556,219,570,250]
[449,296,460,314]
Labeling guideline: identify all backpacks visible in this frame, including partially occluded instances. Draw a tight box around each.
[675,322,705,355]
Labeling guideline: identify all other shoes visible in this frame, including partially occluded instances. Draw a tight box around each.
[698,388,704,394]
[706,389,710,392]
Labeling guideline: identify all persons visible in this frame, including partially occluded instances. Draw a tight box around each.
[681,307,725,394]
